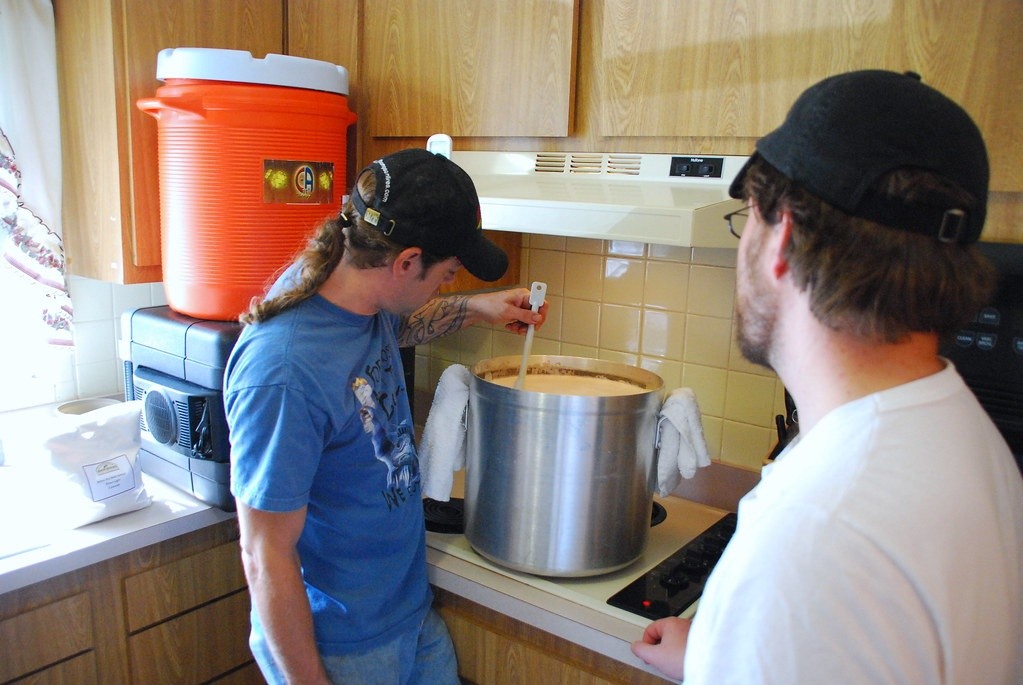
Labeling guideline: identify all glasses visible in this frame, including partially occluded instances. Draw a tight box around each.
[725,201,792,240]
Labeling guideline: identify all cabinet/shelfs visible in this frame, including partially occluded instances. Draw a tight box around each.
[52,0,286,285]
[361,0,991,157]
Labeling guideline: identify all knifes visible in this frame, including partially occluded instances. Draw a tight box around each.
[776,413,787,443]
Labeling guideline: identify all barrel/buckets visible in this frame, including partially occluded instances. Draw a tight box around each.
[463,354,666,578]
[136,46,358,321]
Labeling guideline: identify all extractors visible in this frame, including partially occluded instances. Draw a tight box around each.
[426,133,751,250]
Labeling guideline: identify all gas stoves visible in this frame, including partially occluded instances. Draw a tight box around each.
[421,497,737,648]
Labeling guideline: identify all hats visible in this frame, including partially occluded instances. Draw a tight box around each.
[350,149,509,282]
[728,69,989,246]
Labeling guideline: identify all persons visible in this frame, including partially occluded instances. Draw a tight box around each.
[632,70,1023,685]
[223,147,549,685]
